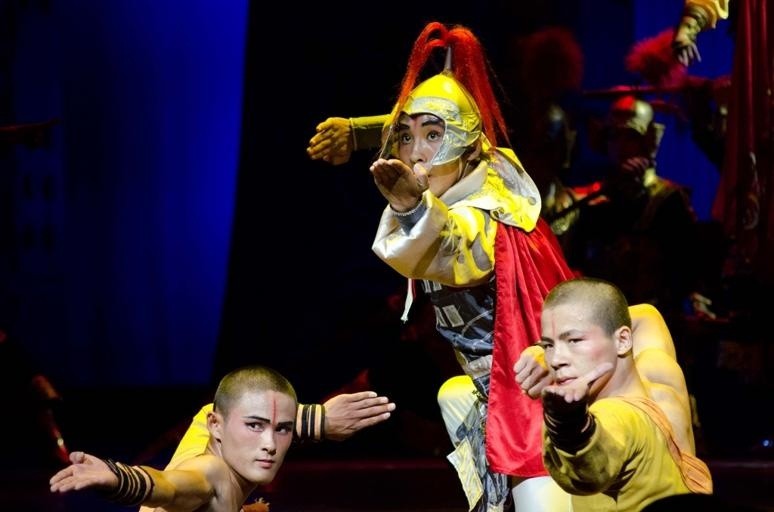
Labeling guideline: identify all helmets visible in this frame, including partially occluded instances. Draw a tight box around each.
[376,47,482,167]
[587,94,664,159]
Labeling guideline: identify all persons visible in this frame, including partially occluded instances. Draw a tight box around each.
[516,105,602,252]
[532,91,715,408]
[671,0,730,69]
[510,276,726,512]
[302,69,587,512]
[45,364,398,512]
